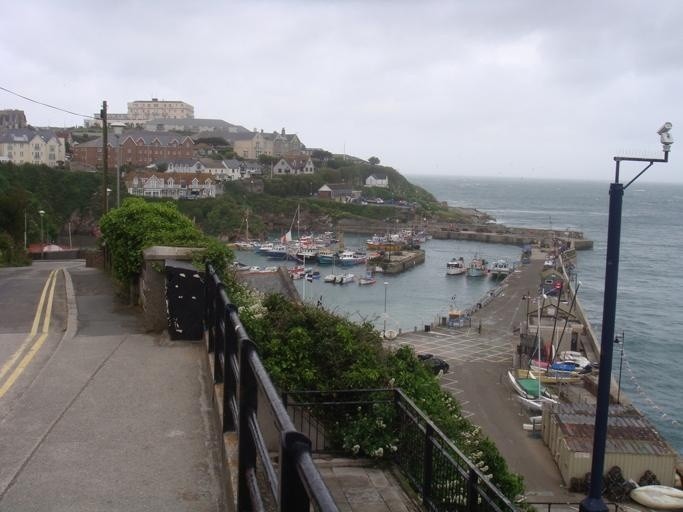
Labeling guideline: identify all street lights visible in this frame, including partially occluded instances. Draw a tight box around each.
[579,122,673,512]
[38,209,46,261]
[107,187,112,214]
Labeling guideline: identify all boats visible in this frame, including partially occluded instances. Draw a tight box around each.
[230,223,433,285]
[447,251,511,278]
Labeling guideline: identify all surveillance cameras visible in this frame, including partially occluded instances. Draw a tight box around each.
[657,122,672,135]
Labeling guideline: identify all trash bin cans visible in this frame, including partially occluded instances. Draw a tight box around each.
[164,259,206,340]
[442,317,446,325]
